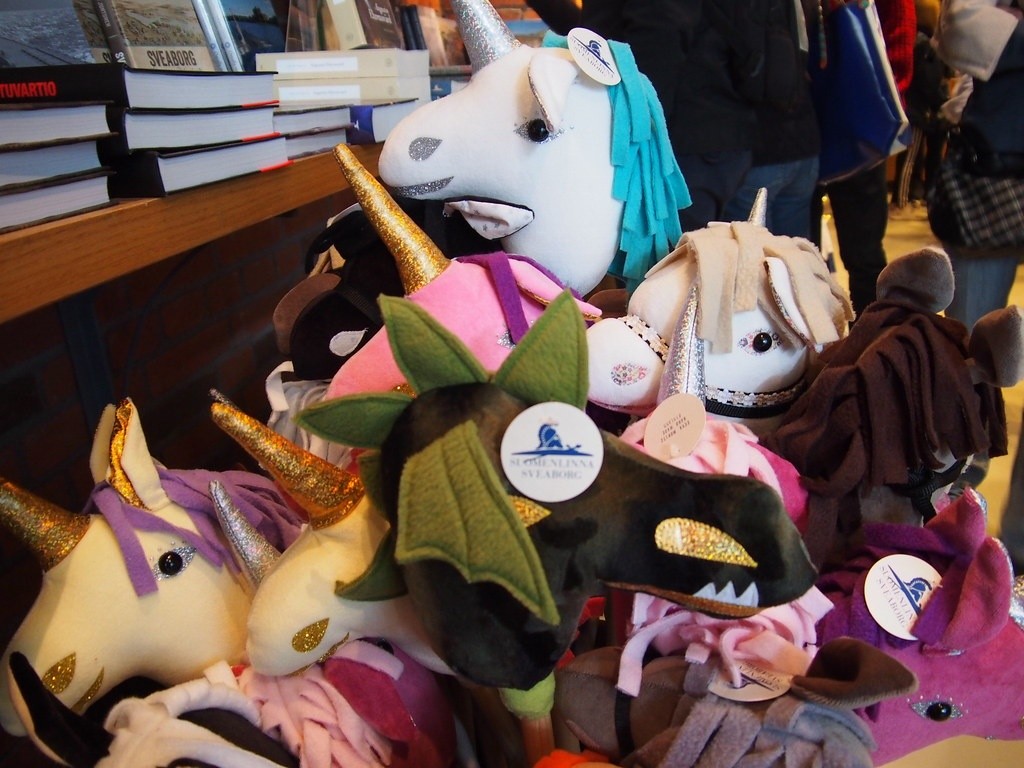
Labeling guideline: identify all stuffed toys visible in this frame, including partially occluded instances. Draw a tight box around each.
[378,0,691,301]
[0,187,1024,768]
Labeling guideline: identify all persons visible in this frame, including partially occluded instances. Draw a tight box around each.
[525,0,1024,333]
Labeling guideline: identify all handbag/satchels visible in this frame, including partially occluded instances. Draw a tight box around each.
[925,159,1024,250]
[797,0,912,184]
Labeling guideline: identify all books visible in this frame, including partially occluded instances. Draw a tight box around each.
[0,0,468,231]
